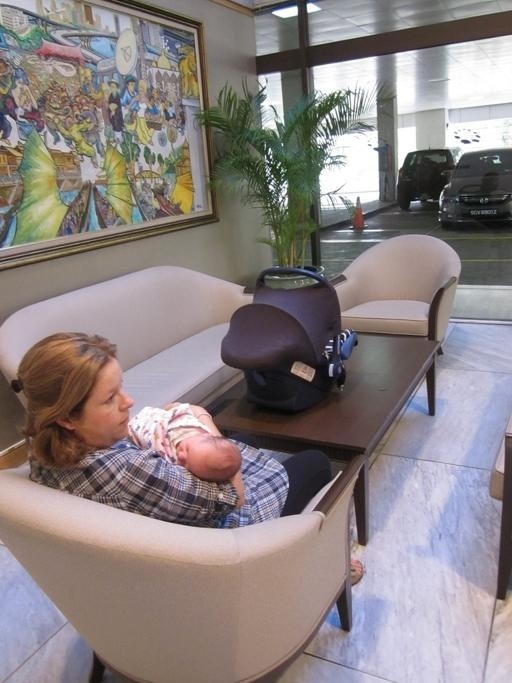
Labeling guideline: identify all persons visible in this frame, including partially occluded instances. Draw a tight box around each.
[11,333,364,590]
[128,402,241,484]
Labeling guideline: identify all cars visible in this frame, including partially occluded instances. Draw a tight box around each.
[437,148,512,228]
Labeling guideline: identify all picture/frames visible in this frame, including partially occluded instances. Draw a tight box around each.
[0,1,219,275]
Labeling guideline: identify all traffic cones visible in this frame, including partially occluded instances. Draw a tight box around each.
[349,196,369,229]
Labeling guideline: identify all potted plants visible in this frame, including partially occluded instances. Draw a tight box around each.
[192,76,398,288]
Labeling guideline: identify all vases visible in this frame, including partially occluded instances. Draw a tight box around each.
[267,270,318,286]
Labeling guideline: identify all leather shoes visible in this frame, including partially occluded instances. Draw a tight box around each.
[347,558,364,585]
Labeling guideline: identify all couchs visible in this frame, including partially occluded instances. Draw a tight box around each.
[1,267,253,424]
[0,432,370,683]
[325,232,461,354]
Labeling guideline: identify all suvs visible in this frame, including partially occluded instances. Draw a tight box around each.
[397,146,455,209]
